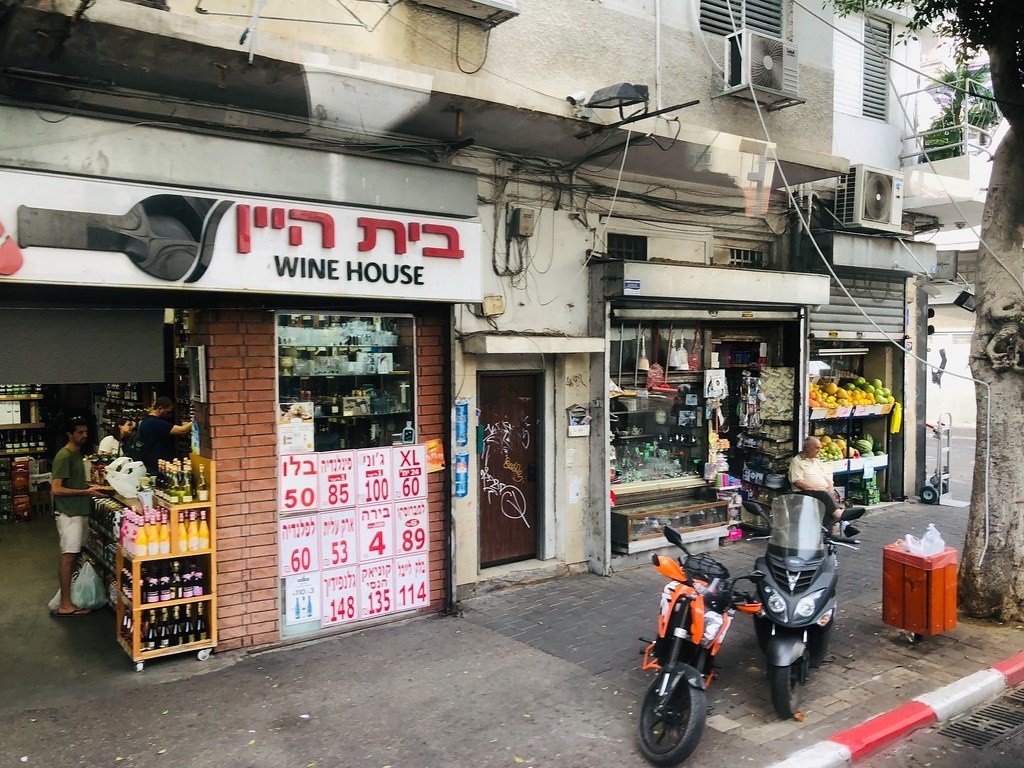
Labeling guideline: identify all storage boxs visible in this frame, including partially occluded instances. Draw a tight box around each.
[833,472,883,505]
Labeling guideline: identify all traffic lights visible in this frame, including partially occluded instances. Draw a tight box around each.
[927,306,936,336]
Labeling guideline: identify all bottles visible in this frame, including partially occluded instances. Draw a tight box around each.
[402,421,415,444]
[0,429,46,450]
[295,597,300,619]
[97,381,149,429]
[175,309,191,454]
[307,595,312,617]
[87,451,210,652]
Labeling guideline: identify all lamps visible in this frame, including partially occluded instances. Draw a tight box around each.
[947,273,977,312]
[670,333,691,372]
[817,347,869,356]
[638,333,649,371]
[585,82,650,111]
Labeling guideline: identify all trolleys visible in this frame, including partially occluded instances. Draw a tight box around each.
[919,411,974,507]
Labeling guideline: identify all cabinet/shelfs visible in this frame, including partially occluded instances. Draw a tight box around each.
[0,384,219,667]
[609,375,712,505]
[740,419,797,536]
[807,380,899,508]
[277,343,411,418]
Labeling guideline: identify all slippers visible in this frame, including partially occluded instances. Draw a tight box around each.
[55,609,91,616]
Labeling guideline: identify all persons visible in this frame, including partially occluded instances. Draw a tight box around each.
[51,418,109,615]
[789,436,866,537]
[98,419,132,456]
[141,397,194,472]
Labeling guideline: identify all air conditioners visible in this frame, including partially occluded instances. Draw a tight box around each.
[833,164,905,230]
[725,29,800,105]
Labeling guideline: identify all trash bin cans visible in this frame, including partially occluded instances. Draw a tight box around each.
[880,537,959,635]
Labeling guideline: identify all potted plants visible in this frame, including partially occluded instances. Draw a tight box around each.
[919,62,1000,163]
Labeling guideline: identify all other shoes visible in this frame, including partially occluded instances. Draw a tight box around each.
[843,524,860,537]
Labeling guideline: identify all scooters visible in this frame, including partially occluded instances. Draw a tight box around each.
[735,492,862,719]
[635,525,767,768]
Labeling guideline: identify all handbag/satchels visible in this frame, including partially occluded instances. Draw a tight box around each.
[106,457,147,498]
[48,572,80,610]
[71,561,107,609]
